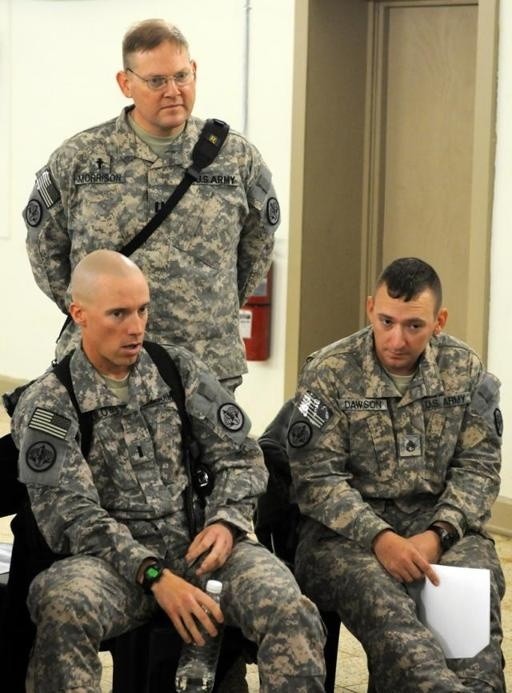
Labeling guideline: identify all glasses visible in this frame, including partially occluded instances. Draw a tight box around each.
[125,59,194,90]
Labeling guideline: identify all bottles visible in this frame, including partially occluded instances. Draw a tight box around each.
[175,578,226,693]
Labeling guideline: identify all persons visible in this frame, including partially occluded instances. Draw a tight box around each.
[285,254,508,690]
[4,249,338,691]
[21,17,282,690]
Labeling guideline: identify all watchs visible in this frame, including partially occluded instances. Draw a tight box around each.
[427,523,454,553]
[141,561,166,596]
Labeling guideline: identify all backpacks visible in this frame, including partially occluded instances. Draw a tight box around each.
[0,339,186,561]
